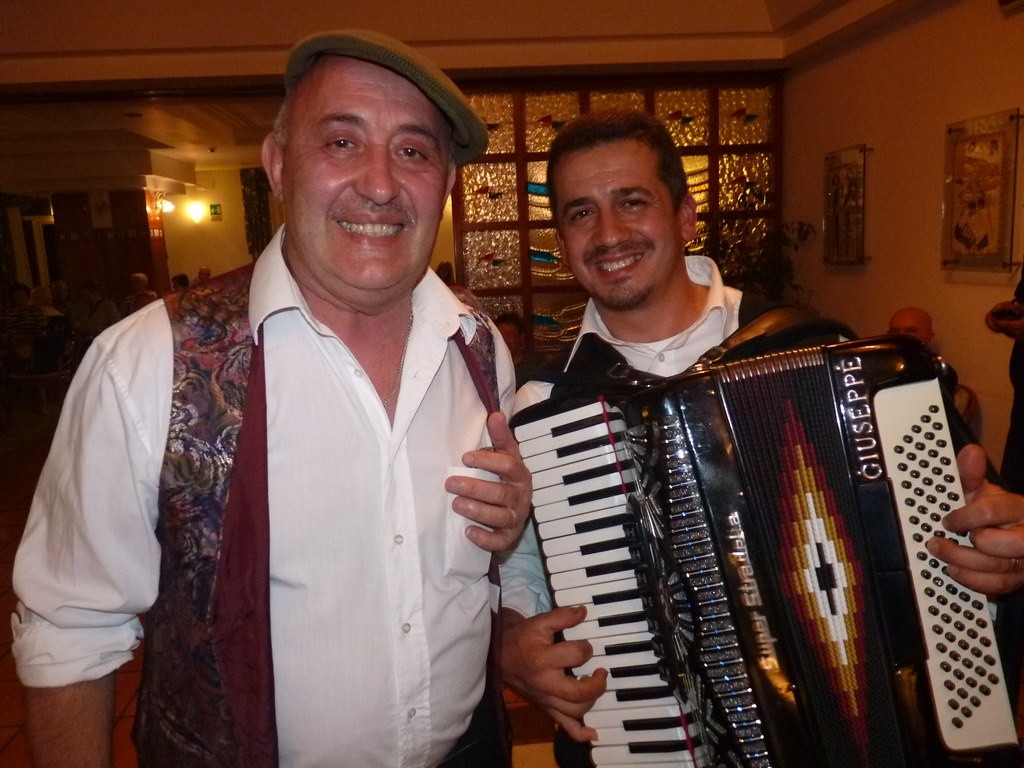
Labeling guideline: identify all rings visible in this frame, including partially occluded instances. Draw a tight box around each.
[1011,559,1019,572]
[509,508,519,527]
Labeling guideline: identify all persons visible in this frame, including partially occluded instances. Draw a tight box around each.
[888,307,974,427]
[0,283,46,371]
[983,266,1024,496]
[71,280,121,355]
[50,282,69,305]
[496,313,533,392]
[500,109,1024,768]
[120,273,157,319]
[11,30,532,768]
[198,266,211,281]
[172,273,189,292]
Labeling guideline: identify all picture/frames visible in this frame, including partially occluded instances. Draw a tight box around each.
[941,107,1018,273]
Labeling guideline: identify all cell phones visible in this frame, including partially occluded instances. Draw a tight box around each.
[991,305,1021,320]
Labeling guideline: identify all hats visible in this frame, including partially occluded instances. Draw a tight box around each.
[283,27,489,168]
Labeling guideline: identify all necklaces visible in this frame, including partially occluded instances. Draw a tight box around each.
[384,309,412,408]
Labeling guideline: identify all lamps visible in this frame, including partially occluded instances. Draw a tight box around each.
[156,198,175,213]
